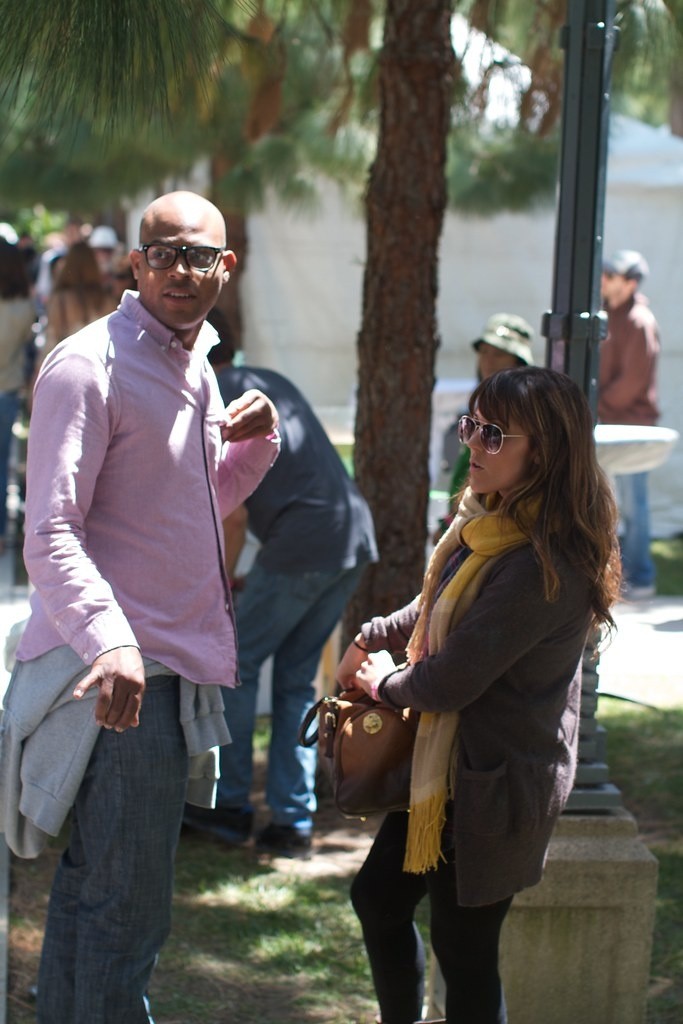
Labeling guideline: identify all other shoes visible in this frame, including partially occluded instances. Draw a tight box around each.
[254,822,314,860]
[179,802,255,842]
[623,583,656,598]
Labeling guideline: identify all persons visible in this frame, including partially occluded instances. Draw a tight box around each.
[334,366,622,1024]
[432,251,659,604]
[0,216,380,856]
[23,191,282,1024]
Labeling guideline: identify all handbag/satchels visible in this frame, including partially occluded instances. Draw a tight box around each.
[299,685,414,815]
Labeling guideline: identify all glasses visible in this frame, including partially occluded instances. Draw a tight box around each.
[138,243,225,272]
[458,416,530,455]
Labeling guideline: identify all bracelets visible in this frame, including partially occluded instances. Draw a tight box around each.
[351,639,369,653]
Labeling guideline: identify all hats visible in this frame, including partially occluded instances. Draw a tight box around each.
[602,250,648,285]
[471,313,536,368]
[89,225,117,252]
